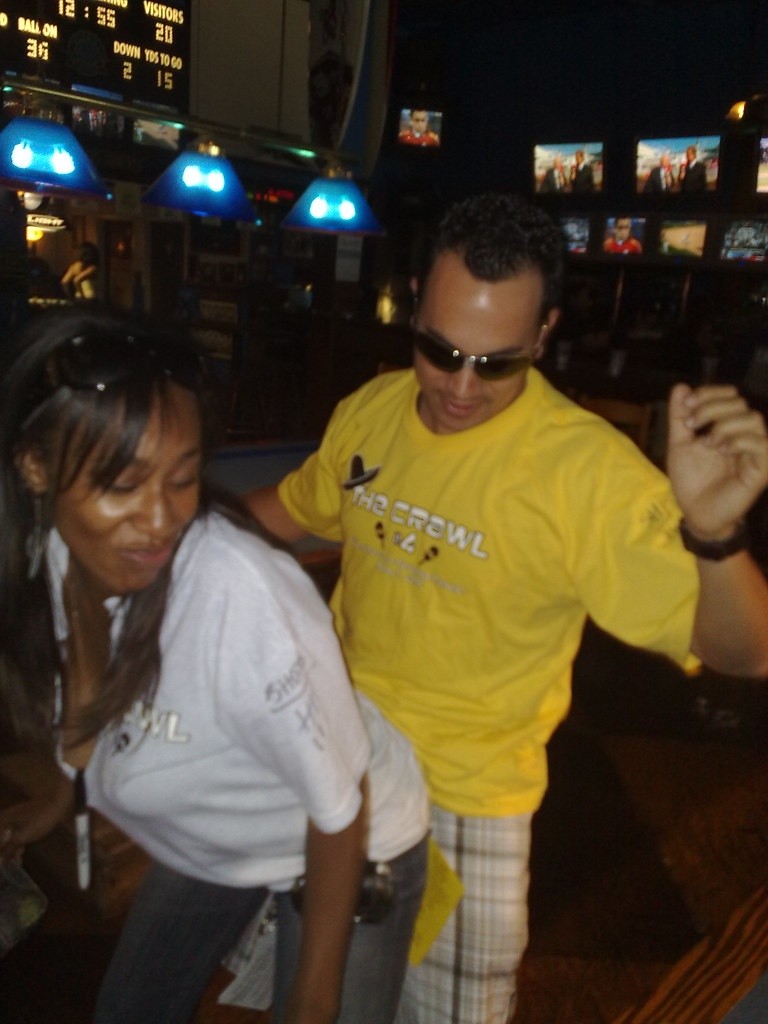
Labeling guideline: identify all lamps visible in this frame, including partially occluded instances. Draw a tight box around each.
[138,132,263,227]
[0,97,106,197]
[278,160,385,237]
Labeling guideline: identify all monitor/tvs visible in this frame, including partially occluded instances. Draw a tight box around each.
[534,134,768,270]
[399,108,443,148]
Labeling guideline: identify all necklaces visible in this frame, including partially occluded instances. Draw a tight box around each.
[70,603,88,698]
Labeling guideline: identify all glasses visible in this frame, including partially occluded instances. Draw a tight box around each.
[15,331,202,444]
[410,292,549,384]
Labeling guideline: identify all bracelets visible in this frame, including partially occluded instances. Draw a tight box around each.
[679,518,745,562]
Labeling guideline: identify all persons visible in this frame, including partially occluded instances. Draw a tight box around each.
[542,147,768,257]
[56,241,98,299]
[236,191,768,1024]
[0,313,432,1024]
[399,110,442,149]
